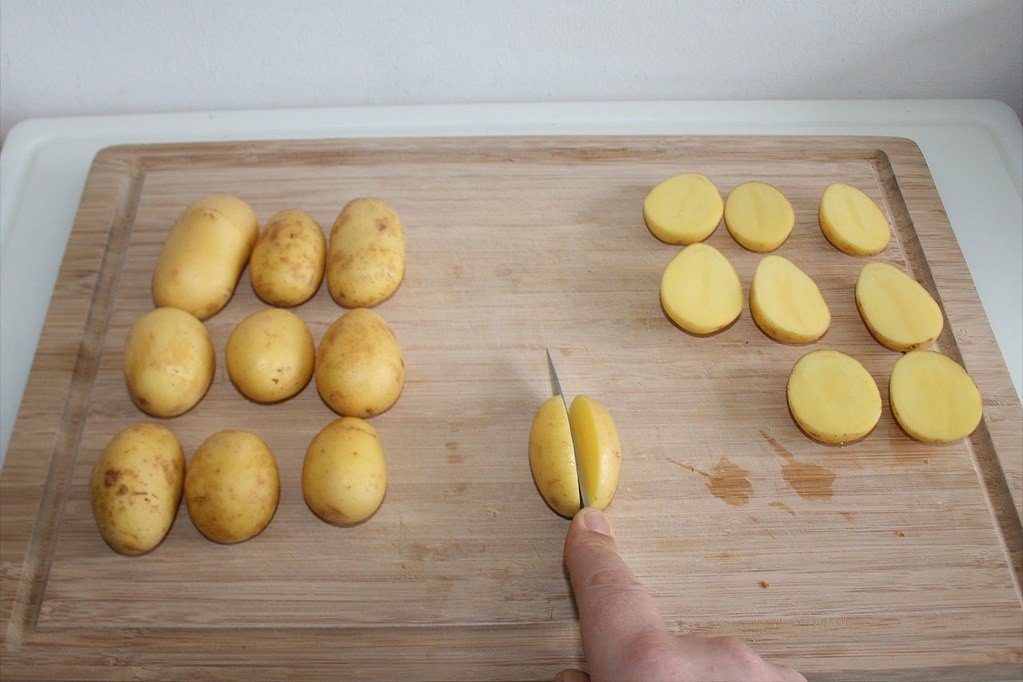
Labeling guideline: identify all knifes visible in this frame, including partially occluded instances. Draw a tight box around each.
[546,346,585,515]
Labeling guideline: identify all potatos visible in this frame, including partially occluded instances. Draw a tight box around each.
[659,242,745,336]
[854,262,943,352]
[316,307,404,419]
[569,394,622,511]
[528,394,580,517]
[250,208,326,308]
[890,350,983,442]
[124,305,215,417]
[786,348,883,445]
[225,309,314,404]
[749,255,831,344]
[89,421,186,557]
[302,417,386,525]
[154,194,260,321]
[644,173,724,245]
[183,429,281,544]
[326,198,406,309]
[725,180,795,252]
[819,182,891,256]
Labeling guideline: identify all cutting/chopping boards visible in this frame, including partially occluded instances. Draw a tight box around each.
[0,139,1023,682]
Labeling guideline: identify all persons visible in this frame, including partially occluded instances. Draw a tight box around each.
[555,506,806,682]
